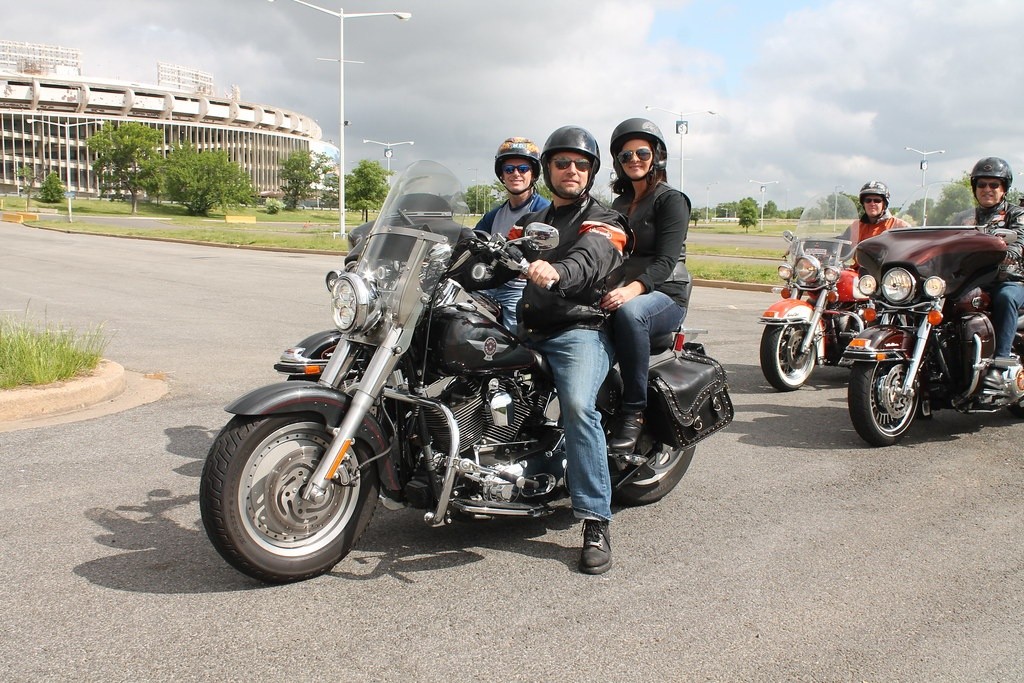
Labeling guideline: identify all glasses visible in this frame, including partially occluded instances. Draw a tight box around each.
[864,198,882,203]
[618,148,651,163]
[503,164,529,173]
[554,157,590,172]
[978,182,1002,189]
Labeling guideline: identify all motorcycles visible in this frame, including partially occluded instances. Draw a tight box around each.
[847,180,1024,446]
[193,158,732,582]
[755,190,878,391]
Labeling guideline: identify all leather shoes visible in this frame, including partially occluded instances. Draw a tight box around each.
[610,413,644,455]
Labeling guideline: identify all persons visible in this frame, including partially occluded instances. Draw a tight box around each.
[837,181,911,243]
[611,119,692,453]
[950,157,1024,395]
[475,125,627,573]
[475,138,551,328]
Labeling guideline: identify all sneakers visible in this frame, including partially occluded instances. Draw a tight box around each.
[580,521,613,574]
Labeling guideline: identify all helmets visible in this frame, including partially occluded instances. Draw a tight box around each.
[494,138,541,174]
[971,156,1013,192]
[610,118,667,157]
[540,126,600,174]
[860,180,890,207]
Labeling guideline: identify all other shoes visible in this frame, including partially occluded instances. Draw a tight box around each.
[982,369,1005,390]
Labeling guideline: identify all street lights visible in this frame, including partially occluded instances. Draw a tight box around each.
[748,179,779,233]
[643,106,715,192]
[467,166,481,217]
[27,118,107,224]
[830,185,846,232]
[904,145,945,224]
[267,0,412,242]
[362,138,415,185]
